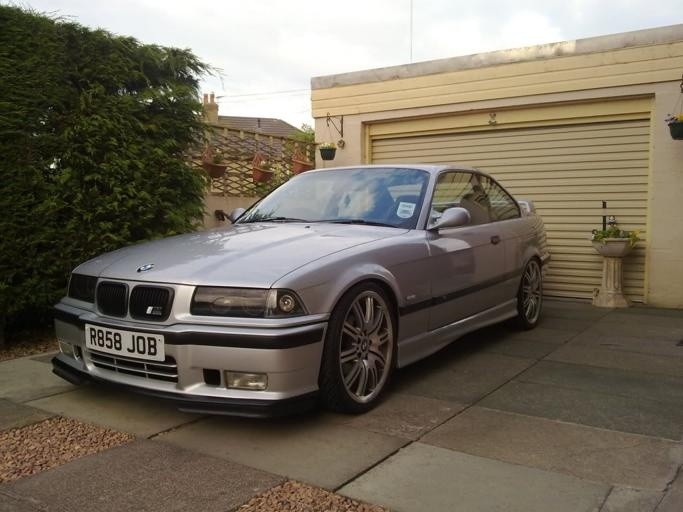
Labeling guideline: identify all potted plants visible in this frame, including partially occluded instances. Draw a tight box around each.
[590,227,641,309]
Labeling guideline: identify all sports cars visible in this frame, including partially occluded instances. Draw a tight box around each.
[47,164,551,418]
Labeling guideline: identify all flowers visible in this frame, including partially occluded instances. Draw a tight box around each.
[213,148,223,163]
[252,150,271,171]
[665,112,683,127]
[319,141,337,149]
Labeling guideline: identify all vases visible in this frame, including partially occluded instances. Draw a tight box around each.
[202,161,228,178]
[320,148,336,161]
[252,167,274,184]
[668,120,683,140]
[291,159,314,175]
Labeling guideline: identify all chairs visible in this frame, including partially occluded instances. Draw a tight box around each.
[384,194,422,226]
[460,193,492,224]
